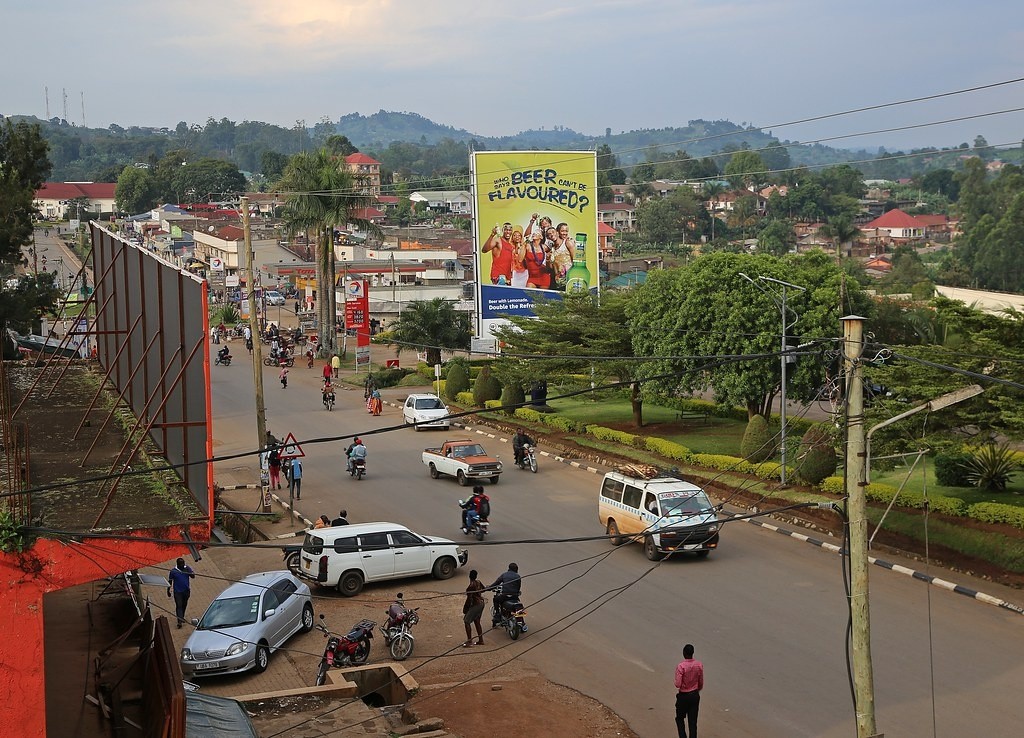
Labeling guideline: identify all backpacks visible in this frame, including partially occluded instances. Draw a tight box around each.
[269,449,280,466]
[478,496,489,519]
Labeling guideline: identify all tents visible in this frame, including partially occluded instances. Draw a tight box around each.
[240,273,258,283]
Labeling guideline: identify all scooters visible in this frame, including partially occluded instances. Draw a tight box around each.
[214,349,233,366]
[282,544,302,577]
[260,333,308,368]
[458,499,491,541]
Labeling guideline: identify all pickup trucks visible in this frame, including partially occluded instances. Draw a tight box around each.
[420,439,504,486]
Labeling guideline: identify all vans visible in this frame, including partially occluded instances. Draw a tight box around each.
[598,465,723,562]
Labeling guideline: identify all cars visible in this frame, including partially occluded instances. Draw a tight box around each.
[262,291,286,306]
[402,393,451,431]
[179,569,314,683]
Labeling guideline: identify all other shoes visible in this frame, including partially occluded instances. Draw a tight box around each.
[278,484,281,489]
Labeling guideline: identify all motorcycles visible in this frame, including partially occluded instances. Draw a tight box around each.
[379,592,420,662]
[343,447,367,480]
[320,387,336,411]
[314,614,378,686]
[485,585,530,640]
[517,444,539,474]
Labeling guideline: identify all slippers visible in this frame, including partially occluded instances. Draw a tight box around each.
[474,642,484,644]
[463,644,473,647]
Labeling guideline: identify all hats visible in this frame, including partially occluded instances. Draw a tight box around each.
[356,440,361,444]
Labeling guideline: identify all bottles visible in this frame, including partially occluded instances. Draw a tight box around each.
[495,222,501,238]
[549,247,556,262]
[525,232,533,243]
[565,233,591,294]
[535,208,540,225]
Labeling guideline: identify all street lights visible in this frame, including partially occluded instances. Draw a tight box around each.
[737,269,806,487]
[394,266,401,337]
[847,385,986,738]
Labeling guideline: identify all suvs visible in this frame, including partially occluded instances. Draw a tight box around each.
[298,521,469,597]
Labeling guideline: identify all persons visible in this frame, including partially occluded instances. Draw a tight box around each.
[283,456,303,501]
[459,486,489,529]
[267,429,284,490]
[446,451,456,458]
[512,428,537,464]
[332,510,350,528]
[462,570,487,647]
[648,497,668,512]
[674,644,703,738]
[295,298,386,416]
[314,515,331,529]
[485,563,528,633]
[482,212,576,292]
[29,212,294,389]
[167,557,196,629]
[346,436,367,473]
